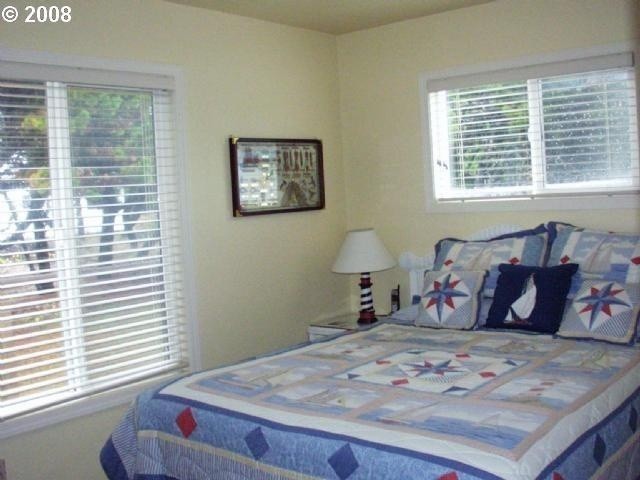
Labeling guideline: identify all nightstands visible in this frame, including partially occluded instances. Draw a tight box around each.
[307,313,387,341]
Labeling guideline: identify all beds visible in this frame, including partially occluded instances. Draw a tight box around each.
[100,225,640,480]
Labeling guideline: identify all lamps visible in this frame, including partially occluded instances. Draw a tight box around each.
[331,228,399,326]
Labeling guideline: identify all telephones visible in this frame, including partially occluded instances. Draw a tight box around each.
[391,284,400,313]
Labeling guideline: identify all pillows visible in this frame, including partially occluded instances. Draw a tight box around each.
[414,221,640,346]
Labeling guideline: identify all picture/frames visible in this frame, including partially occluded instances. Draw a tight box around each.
[229,135,325,217]
